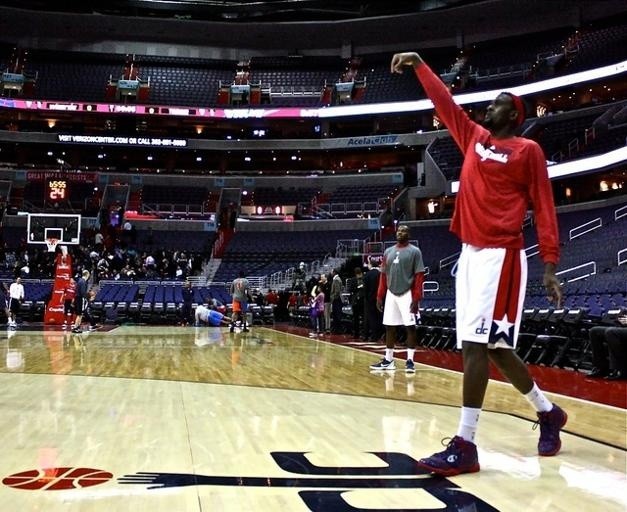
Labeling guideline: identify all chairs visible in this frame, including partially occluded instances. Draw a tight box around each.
[0,16,626,380]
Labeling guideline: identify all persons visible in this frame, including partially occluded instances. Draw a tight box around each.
[391,50,568,477]
[587,314,627,379]
[349,267,365,340]
[181,266,346,337]
[1,270,103,334]
[1,203,210,283]
[361,259,382,341]
[369,224,426,373]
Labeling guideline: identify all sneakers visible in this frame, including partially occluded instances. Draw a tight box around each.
[89,326,102,330]
[406,360,415,373]
[418,436,480,476]
[62,323,82,333]
[536,403,567,456]
[309,329,330,338]
[230,321,249,332]
[369,360,396,369]
[7,321,17,326]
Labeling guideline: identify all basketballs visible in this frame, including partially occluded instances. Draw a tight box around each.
[49,318,55,325]
